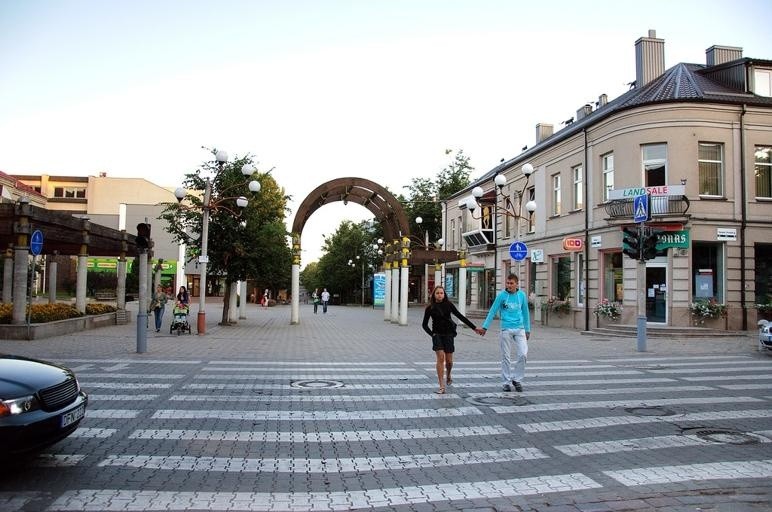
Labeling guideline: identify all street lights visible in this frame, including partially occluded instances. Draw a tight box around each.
[409,215,444,306]
[174,148,261,335]
[347,255,373,306]
[373,239,385,256]
[467,164,537,291]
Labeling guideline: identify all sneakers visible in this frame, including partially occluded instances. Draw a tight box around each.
[512,380,522,392]
[503,384,511,392]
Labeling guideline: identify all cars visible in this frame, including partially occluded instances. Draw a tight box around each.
[0,352,87,453]
[758,321,772,349]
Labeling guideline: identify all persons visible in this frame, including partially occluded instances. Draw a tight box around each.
[312,287,319,314]
[150,284,168,332]
[481,273,530,391]
[263,288,269,311]
[422,285,480,394]
[321,287,331,314]
[177,285,192,332]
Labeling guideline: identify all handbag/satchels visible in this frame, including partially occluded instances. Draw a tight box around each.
[444,318,457,338]
[150,300,158,310]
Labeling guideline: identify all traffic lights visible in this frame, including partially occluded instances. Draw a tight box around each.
[641,232,659,259]
[622,227,641,262]
[134,222,151,248]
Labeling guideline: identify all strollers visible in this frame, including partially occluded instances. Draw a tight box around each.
[169,302,192,335]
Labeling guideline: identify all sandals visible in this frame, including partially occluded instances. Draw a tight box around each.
[437,388,445,394]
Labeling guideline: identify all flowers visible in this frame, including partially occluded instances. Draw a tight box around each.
[541,295,569,314]
[593,298,624,322]
[688,296,728,327]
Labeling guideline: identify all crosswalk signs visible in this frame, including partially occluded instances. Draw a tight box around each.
[632,194,649,223]
